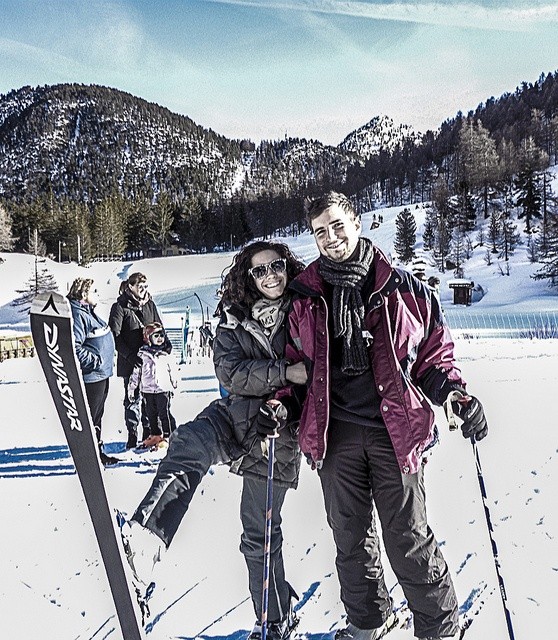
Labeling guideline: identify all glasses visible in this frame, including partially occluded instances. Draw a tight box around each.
[137,284,149,290]
[154,333,165,338]
[246,256,288,282]
[88,288,99,294]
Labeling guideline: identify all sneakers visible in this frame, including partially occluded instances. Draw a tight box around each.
[419,621,464,640]
[335,612,397,639]
[247,613,299,640]
[115,506,163,586]
[144,434,157,447]
[155,437,167,450]
[100,452,120,467]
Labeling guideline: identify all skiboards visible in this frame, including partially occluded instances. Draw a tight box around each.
[27,291,299,640]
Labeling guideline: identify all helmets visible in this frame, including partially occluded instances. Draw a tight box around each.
[142,322,163,347]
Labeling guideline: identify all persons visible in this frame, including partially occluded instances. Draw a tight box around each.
[65,276,119,468]
[127,320,182,446]
[108,273,172,451]
[115,238,305,640]
[252,191,488,640]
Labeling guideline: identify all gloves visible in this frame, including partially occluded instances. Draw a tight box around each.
[129,395,134,403]
[162,340,173,354]
[437,381,488,441]
[127,353,143,369]
[255,401,281,442]
